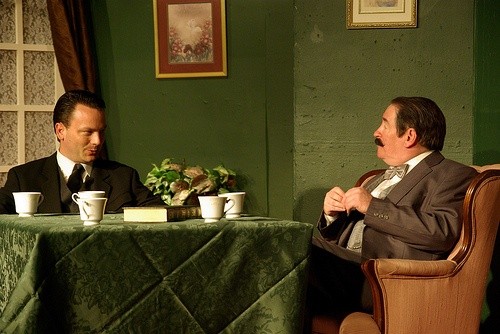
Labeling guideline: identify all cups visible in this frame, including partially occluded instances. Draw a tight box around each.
[198,196,235,223]
[12,192,45,218]
[72,191,107,226]
[218,192,245,219]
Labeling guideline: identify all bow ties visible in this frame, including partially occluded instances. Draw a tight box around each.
[383,164,409,180]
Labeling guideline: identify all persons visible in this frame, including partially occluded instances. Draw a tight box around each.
[0,90,167,215]
[295,97,478,334]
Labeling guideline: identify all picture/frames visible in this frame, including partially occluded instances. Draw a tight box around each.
[345,0,418,30]
[152,0,227,79]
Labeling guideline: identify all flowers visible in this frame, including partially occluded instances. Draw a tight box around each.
[144,158,236,206]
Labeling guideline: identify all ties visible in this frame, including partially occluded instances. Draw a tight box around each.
[66,163,85,194]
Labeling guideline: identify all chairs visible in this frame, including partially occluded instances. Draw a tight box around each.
[312,164,500,334]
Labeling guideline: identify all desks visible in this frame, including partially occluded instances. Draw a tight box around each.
[0,213,315,334]
